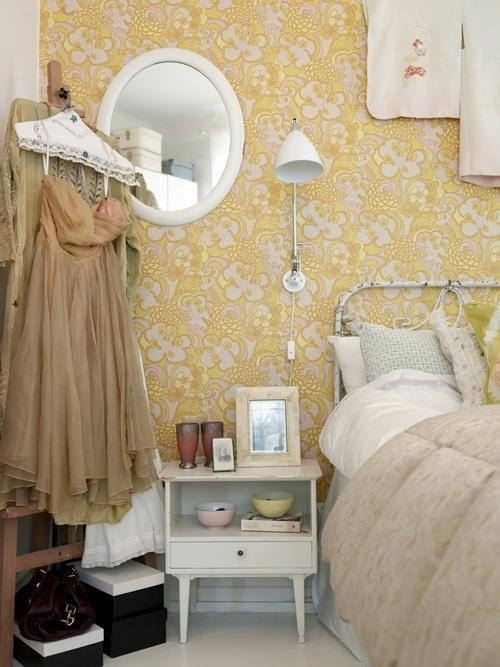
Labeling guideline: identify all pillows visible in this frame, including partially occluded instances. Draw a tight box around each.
[327,333,366,397]
[461,300,500,406]
[428,311,485,406]
[360,319,452,383]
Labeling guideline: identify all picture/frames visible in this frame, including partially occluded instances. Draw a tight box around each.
[236,385,301,468]
[210,436,236,473]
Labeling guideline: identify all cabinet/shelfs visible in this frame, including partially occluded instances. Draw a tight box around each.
[132,165,199,213]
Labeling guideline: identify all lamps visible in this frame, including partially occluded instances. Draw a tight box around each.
[271,115,325,294]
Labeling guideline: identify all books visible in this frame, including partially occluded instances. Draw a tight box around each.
[240,509,304,533]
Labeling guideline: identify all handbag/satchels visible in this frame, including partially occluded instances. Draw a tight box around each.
[14,564,94,642]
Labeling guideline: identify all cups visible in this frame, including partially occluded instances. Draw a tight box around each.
[199,419,226,468]
[177,421,200,471]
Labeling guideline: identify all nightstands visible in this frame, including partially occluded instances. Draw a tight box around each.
[159,459,325,647]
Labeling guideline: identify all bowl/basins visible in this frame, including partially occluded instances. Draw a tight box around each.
[250,489,294,517]
[192,499,238,530]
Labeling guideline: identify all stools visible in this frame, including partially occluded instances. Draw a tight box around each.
[1,504,84,665]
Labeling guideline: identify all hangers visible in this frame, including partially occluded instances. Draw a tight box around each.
[13,87,141,187]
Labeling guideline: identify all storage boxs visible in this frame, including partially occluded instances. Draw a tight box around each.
[96,608,169,658]
[13,616,105,667]
[70,559,165,618]
[161,158,197,182]
[111,127,164,156]
[121,147,162,173]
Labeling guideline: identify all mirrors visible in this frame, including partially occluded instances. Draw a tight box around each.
[95,46,244,226]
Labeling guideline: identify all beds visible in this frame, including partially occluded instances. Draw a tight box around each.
[312,278,500,667]
